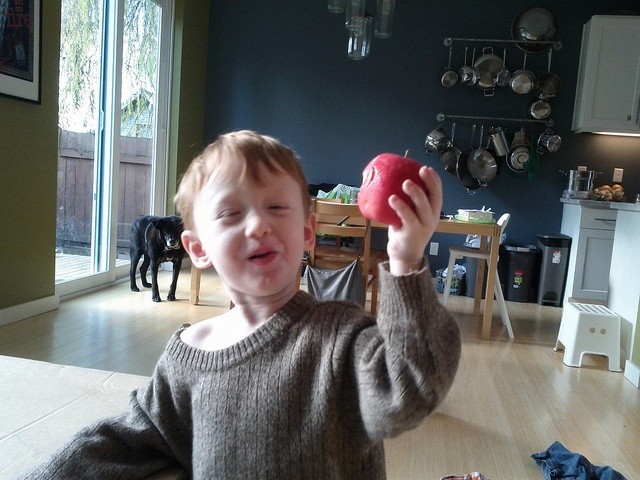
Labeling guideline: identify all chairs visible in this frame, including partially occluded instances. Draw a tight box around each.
[311,198,387,315]
[319,198,344,246]
[441,212,515,339]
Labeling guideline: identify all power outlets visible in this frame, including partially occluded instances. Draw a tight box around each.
[612,168,623,183]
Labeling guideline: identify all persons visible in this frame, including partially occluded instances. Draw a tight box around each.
[10,129,461,480]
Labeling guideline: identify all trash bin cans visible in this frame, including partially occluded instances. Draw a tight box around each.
[503,243,540,305]
[464,244,497,298]
[536,233,572,307]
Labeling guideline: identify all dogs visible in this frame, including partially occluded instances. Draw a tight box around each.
[129,215,186,302]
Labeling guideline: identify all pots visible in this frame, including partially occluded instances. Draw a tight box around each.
[533,46,561,101]
[510,5,562,54]
[457,46,473,84]
[441,122,462,176]
[536,128,562,155]
[474,45,504,97]
[455,122,480,190]
[439,46,459,88]
[508,49,536,97]
[424,122,449,156]
[505,144,532,176]
[493,47,511,88]
[526,99,553,122]
[466,125,498,187]
[558,166,604,199]
[487,126,511,157]
[465,47,481,88]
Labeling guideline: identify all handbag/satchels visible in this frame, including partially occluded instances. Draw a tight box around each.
[305,257,365,311]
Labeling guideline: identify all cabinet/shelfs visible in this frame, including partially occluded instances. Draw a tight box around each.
[561,204,617,305]
[444,37,562,51]
[571,14,640,136]
[436,113,556,128]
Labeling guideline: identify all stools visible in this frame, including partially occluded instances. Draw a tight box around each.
[552,303,622,372]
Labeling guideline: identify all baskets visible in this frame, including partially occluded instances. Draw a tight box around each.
[435,263,466,296]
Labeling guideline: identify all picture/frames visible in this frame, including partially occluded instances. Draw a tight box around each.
[1,1,43,105]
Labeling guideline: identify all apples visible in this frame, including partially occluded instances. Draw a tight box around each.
[357,150,431,228]
